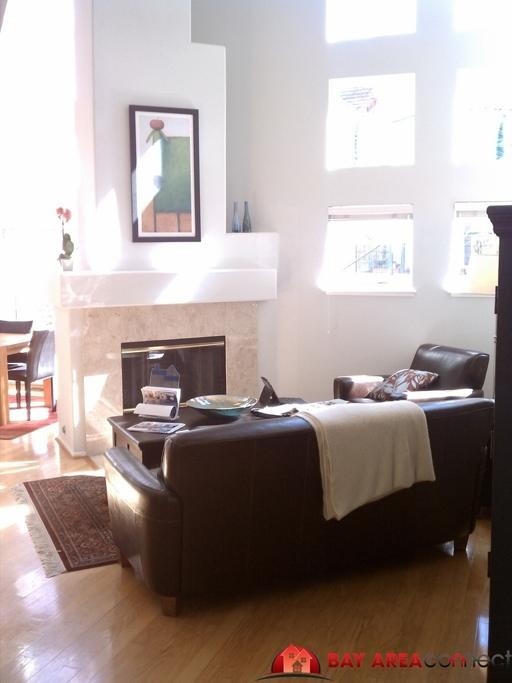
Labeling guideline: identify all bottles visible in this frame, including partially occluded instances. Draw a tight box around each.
[231,200,252,232]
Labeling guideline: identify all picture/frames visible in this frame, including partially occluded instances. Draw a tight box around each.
[127,105,201,241]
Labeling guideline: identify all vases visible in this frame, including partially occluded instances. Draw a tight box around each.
[60,258,74,270]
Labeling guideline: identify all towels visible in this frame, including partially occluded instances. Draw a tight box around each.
[294,401,436,521]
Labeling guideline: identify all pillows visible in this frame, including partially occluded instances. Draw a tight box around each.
[366,368,439,402]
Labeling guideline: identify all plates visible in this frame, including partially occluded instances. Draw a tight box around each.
[186,394,258,417]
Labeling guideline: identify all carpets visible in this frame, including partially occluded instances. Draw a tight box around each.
[0,380,59,439]
[11,468,121,578]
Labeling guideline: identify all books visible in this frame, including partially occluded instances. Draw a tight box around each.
[125,421,186,435]
[133,386,182,422]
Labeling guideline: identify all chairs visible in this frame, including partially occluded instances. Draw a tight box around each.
[334,343,490,403]
[0,319,56,422]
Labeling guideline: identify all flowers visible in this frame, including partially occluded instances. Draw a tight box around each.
[57,207,74,256]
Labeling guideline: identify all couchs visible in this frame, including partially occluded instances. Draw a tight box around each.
[104,397,495,617]
